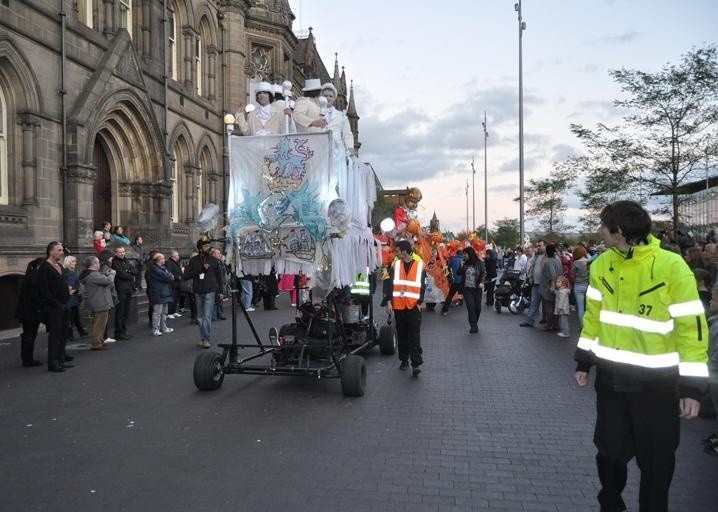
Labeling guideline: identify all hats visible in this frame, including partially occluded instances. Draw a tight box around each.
[301,78,337,96]
[254,81,282,98]
[196,236,212,249]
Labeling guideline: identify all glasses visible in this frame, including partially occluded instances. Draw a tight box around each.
[387,314,393,326]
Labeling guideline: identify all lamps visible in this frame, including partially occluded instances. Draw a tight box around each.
[243,103,255,117]
[222,113,236,133]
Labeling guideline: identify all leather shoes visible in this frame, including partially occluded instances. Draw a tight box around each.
[22,359,42,367]
[48,355,74,371]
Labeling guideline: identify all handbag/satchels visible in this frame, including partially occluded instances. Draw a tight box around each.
[180,261,194,293]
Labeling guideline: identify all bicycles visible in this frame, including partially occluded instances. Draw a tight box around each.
[507,278,529,314]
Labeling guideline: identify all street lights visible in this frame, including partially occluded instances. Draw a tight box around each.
[469,156,477,235]
[512,0,529,247]
[481,110,491,246]
[702,145,711,233]
[464,177,471,235]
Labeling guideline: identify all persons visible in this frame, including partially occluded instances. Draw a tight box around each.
[703,284,718,455]
[145,239,231,348]
[350,272,377,315]
[655,223,717,307]
[441,247,488,333]
[494,239,609,337]
[80,221,144,350]
[574,200,710,512]
[239,265,309,312]
[381,241,426,377]
[238,77,351,143]
[20,241,88,370]
[484,249,496,307]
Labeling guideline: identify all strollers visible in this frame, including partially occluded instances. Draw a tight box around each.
[494,268,527,317]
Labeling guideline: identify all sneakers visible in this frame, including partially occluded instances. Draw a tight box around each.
[197,338,212,348]
[217,312,227,320]
[399,359,409,371]
[412,365,421,377]
[519,319,570,338]
[151,312,182,337]
[96,332,130,350]
[700,432,718,454]
[245,307,256,312]
